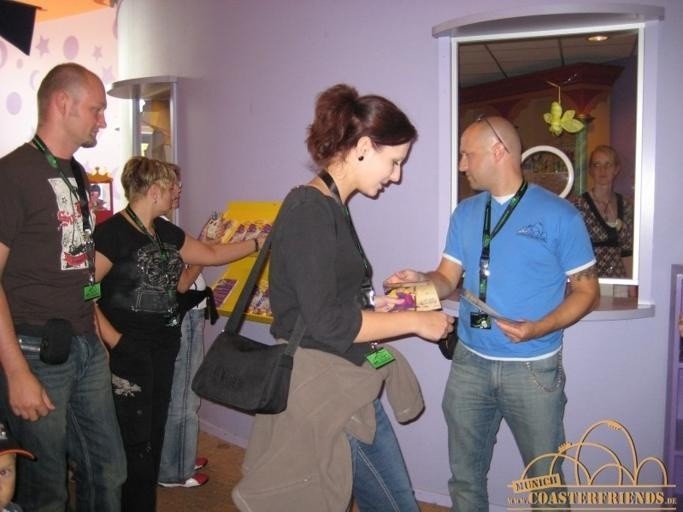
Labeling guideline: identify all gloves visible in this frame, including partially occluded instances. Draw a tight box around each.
[112,334,152,375]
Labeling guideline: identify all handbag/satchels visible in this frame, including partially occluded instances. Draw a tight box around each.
[191,330,293,416]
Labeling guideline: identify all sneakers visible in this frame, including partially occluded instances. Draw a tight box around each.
[158,473,209,489]
[192,458,208,470]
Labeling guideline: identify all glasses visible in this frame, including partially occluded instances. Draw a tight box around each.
[477,112,509,155]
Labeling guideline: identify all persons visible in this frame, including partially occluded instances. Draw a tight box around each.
[383,117,601,512]
[0,63,128,512]
[267,84,453,512]
[94,157,272,512]
[569,144,633,277]
[0,423,34,512]
[153,162,226,490]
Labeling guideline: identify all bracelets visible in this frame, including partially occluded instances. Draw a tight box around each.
[253,238,259,253]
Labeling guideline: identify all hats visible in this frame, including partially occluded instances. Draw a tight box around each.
[1,423,36,461]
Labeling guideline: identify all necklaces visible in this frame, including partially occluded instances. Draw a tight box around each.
[590,186,617,222]
